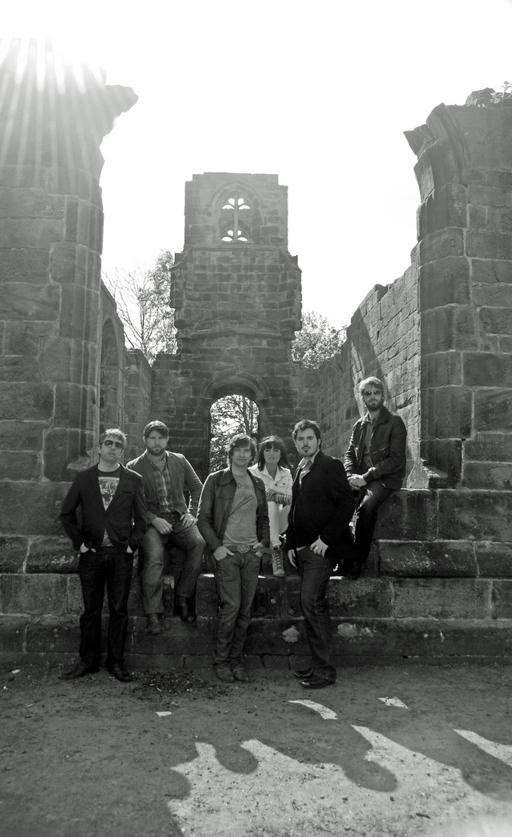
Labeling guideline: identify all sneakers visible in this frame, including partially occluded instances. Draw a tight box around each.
[214,659,250,682]
[273,548,285,577]
[332,560,362,580]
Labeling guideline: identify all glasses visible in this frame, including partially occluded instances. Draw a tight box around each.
[364,391,382,395]
[101,440,123,448]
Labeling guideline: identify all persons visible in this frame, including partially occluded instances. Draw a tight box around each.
[248,434,294,578]
[344,376,407,577]
[284,418,355,690]
[196,433,270,682]
[126,420,206,635]
[58,428,147,680]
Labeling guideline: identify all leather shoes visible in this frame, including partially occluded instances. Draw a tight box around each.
[107,664,131,682]
[294,666,332,688]
[175,596,197,623]
[149,612,162,635]
[61,661,98,679]
